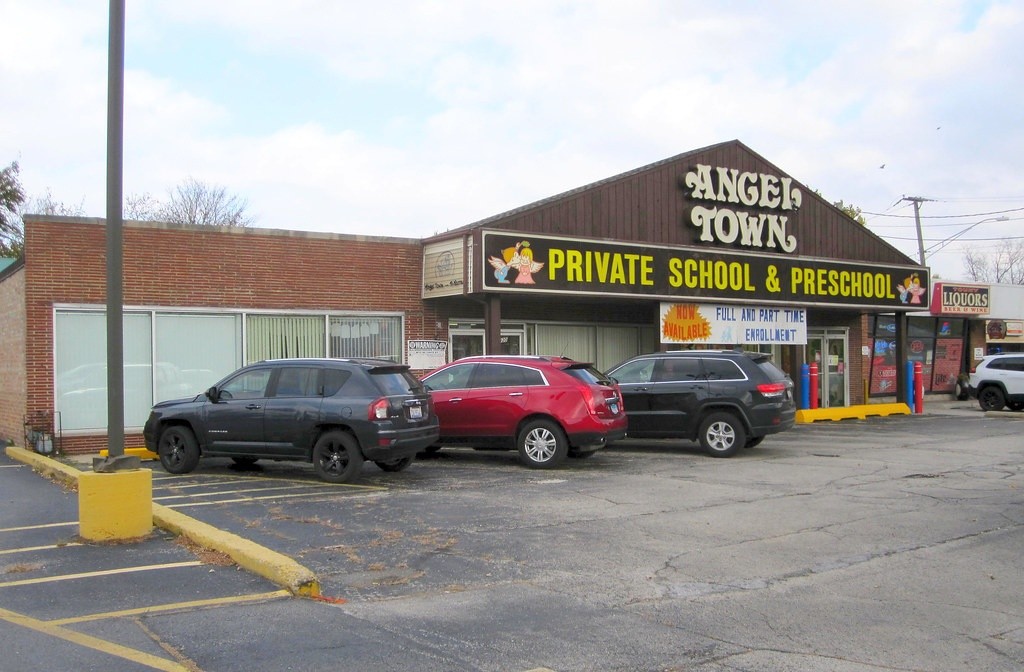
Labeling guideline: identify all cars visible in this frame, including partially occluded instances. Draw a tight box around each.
[326,412,340,424]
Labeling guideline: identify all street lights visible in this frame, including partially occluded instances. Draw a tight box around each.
[918,216,1010,267]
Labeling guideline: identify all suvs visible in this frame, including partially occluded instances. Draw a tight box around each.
[422,355,628,469]
[61,364,155,428]
[177,370,212,395]
[143,358,440,483]
[967,352,1024,411]
[818,358,844,404]
[63,363,128,388]
[603,349,797,458]
